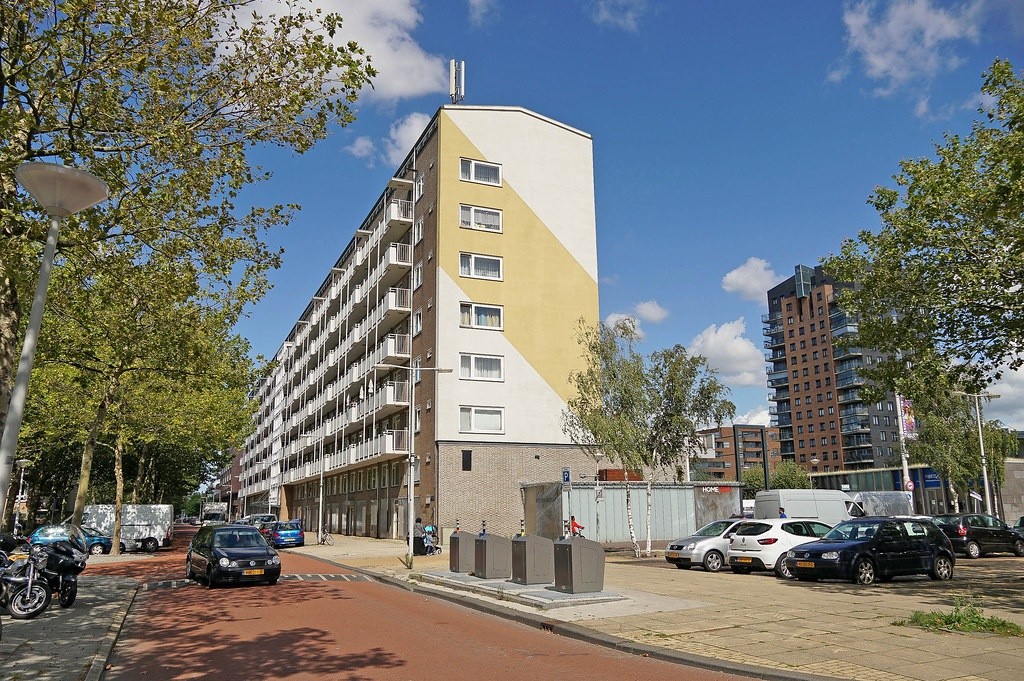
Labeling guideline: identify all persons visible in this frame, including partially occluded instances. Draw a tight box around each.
[425,531,438,556]
[214,534,258,547]
[571,516,584,536]
[778,507,786,518]
[321,523,328,545]
[414,517,426,556]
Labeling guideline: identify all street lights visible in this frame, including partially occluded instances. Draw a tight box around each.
[12,459,33,535]
[299,434,332,544]
[222,485,233,523]
[256,461,273,514]
[197,494,214,523]
[949,385,1001,526]
[372,362,454,569]
[234,476,247,517]
[212,490,221,502]
[0,162,111,528]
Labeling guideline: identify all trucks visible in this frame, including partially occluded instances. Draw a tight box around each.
[844,491,915,517]
[752,487,868,519]
[57,503,174,552]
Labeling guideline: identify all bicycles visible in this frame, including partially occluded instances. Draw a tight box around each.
[573,527,585,539]
[316,523,335,546]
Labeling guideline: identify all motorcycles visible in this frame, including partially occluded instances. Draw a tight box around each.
[0,534,90,620]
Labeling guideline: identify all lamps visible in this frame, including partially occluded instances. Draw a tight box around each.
[840,483,851,491]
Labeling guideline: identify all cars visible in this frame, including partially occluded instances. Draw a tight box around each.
[931,512,1024,559]
[185,524,283,589]
[864,514,948,538]
[784,515,956,585]
[724,517,850,577]
[183,507,305,549]
[25,522,138,555]
[662,513,762,574]
